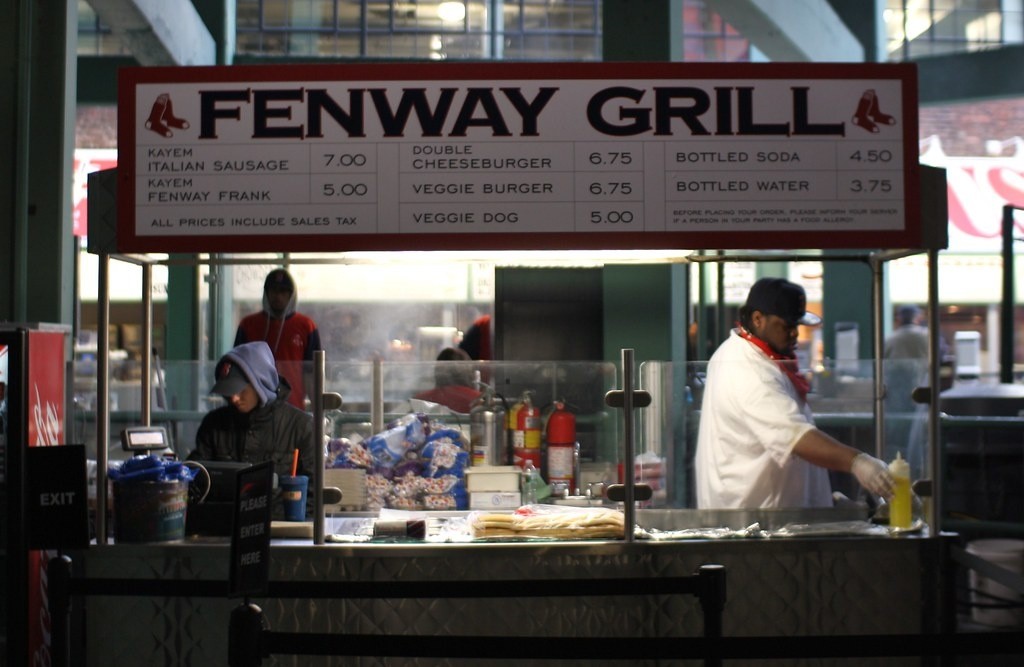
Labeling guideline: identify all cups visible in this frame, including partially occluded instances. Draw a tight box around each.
[887,450,912,527]
[279,475,309,521]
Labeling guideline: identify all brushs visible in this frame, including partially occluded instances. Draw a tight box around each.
[372,519,426,540]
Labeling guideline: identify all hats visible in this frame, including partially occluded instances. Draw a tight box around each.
[747,278,821,326]
[265,270,292,291]
[210,356,250,394]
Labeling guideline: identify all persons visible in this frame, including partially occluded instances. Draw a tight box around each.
[412,315,491,413]
[883,305,950,444]
[694,278,898,508]
[233,269,321,411]
[185,342,316,510]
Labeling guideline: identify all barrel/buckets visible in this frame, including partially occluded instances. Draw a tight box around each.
[111,477,187,544]
[965,539,1024,627]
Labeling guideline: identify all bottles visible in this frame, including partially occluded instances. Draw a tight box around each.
[522,459,537,506]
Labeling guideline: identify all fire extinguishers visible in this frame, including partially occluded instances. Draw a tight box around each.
[508,391,542,470]
[540,396,576,496]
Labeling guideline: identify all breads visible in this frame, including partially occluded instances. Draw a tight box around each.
[474,515,623,538]
